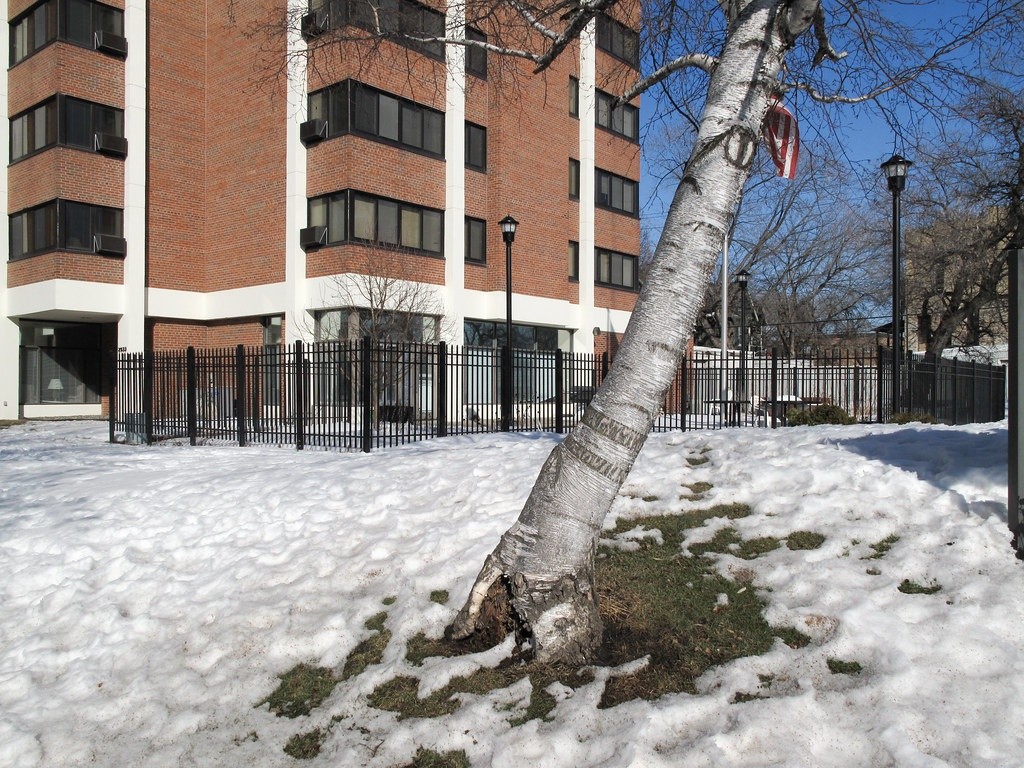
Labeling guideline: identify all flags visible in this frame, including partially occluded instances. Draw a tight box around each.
[759,91,799,182]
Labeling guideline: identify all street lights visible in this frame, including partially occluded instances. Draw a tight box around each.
[882,151,913,420]
[735,267,752,414]
[497,212,519,427]
